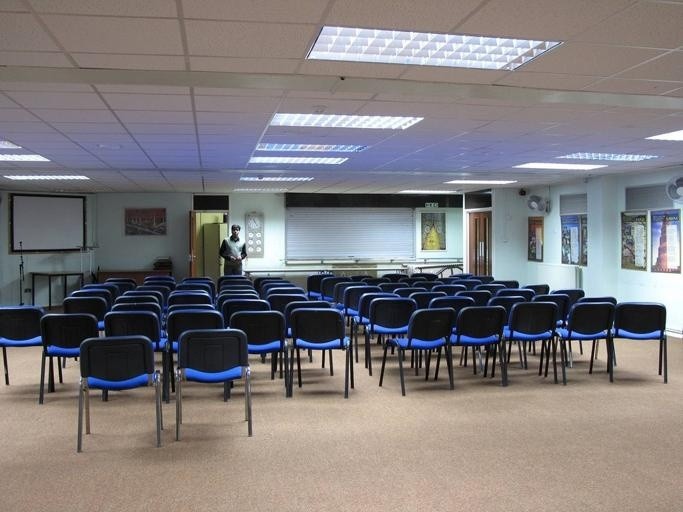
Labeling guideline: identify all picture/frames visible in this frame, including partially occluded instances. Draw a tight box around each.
[120,204,169,239]
[419,211,448,252]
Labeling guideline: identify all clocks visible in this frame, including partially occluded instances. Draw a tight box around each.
[244,212,264,258]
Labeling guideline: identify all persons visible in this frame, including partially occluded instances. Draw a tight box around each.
[219,224,248,275]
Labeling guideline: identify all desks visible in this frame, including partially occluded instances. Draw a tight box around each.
[28,272,84,310]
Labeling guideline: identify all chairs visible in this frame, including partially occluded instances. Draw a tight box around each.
[63,276,336,379]
[77,336,162,453]
[545,303,615,385]
[589,302,669,383]
[178,328,254,444]
[491,302,557,385]
[306,274,618,378]
[435,307,507,387]
[1,305,52,392]
[378,308,456,397]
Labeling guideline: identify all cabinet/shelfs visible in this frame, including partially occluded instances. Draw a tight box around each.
[202,221,227,276]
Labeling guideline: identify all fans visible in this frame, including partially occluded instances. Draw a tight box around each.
[665,172,682,207]
[525,194,552,215]
